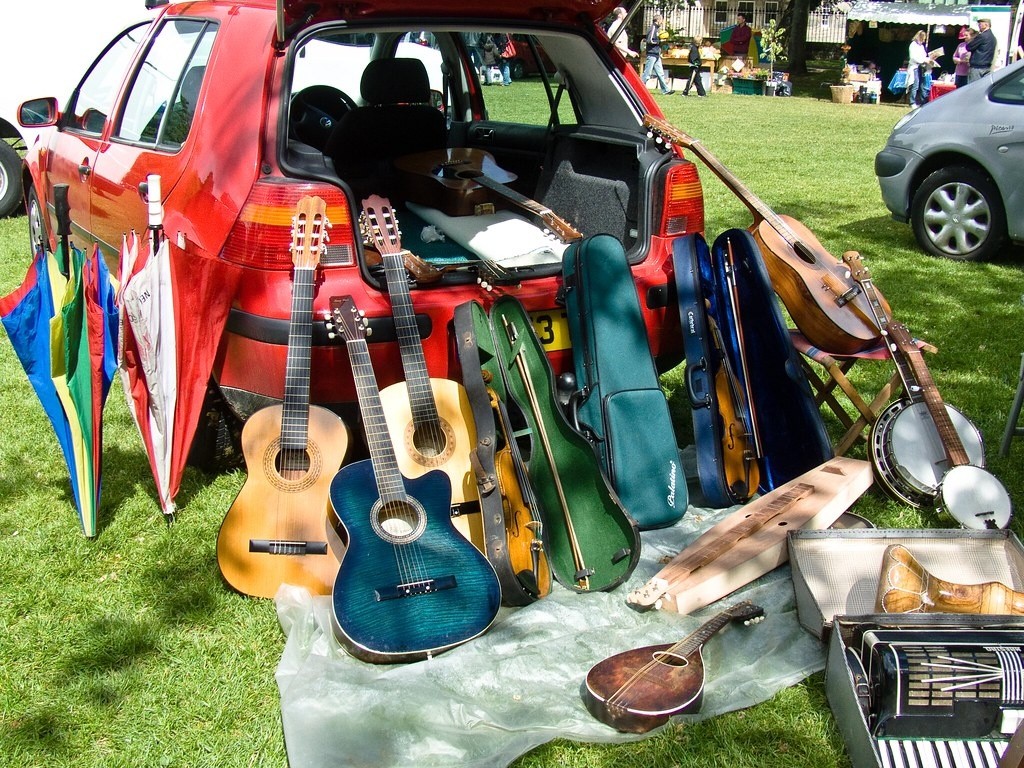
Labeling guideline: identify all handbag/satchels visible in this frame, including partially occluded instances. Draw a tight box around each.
[500,41,516,57]
[918,63,928,77]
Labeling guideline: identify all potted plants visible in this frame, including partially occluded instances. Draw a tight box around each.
[830,82,853,104]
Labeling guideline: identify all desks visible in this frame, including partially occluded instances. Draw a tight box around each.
[887,71,907,104]
[658,57,716,92]
[929,83,956,101]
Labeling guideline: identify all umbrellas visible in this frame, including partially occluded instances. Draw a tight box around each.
[120,175,243,526]
[0,182,121,542]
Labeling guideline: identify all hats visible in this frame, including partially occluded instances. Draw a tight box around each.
[977,18,991,23]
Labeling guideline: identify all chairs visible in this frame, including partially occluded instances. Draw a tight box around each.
[324,57,450,169]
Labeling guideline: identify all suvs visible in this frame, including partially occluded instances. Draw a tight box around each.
[0,1,468,222]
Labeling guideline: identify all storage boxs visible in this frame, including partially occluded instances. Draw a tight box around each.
[784,529,1024,768]
[731,78,764,94]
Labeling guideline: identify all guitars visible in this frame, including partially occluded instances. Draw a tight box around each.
[353,190,489,556]
[837,250,989,514]
[317,299,508,663]
[360,235,446,285]
[394,146,589,242]
[886,319,1015,532]
[640,108,894,356]
[214,188,350,603]
[582,598,767,734]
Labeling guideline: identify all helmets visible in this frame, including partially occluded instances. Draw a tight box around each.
[659,30,669,40]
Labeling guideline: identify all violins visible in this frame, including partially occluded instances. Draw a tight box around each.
[478,366,552,600]
[706,296,762,499]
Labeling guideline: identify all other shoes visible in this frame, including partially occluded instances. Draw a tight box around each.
[663,91,675,95]
[681,93,690,96]
[909,103,918,111]
[698,95,708,98]
[482,81,491,86]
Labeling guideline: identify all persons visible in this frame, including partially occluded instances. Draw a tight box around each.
[908,30,936,108]
[730,13,751,55]
[608,7,639,58]
[462,32,512,87]
[966,19,997,84]
[641,15,674,95]
[703,40,713,48]
[953,29,978,89]
[682,36,706,97]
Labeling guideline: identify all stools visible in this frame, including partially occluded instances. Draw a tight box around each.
[786,326,938,456]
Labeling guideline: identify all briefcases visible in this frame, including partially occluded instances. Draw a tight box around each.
[783,528,1024,768]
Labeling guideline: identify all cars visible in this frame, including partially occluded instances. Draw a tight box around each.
[14,0,705,471]
[872,56,1024,261]
